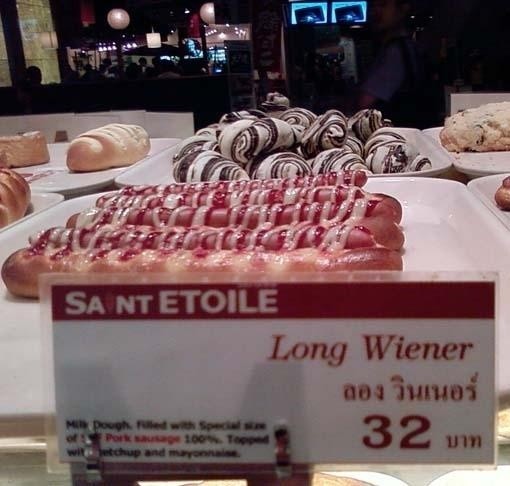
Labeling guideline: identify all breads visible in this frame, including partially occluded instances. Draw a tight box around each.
[439,101,510,154]
[66,124,151,172]
[2,168,406,299]
[2,131,50,168]
[2,168,32,227]
[495,174,509,209]
[171,91,432,182]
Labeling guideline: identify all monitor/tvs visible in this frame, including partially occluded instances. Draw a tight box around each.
[283,0,329,26]
[329,0,369,26]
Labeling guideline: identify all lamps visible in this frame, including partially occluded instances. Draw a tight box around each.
[39,22,58,51]
[144,25,162,50]
[106,8,130,29]
[200,2,216,25]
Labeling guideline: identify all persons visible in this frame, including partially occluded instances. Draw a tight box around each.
[25,50,179,81]
